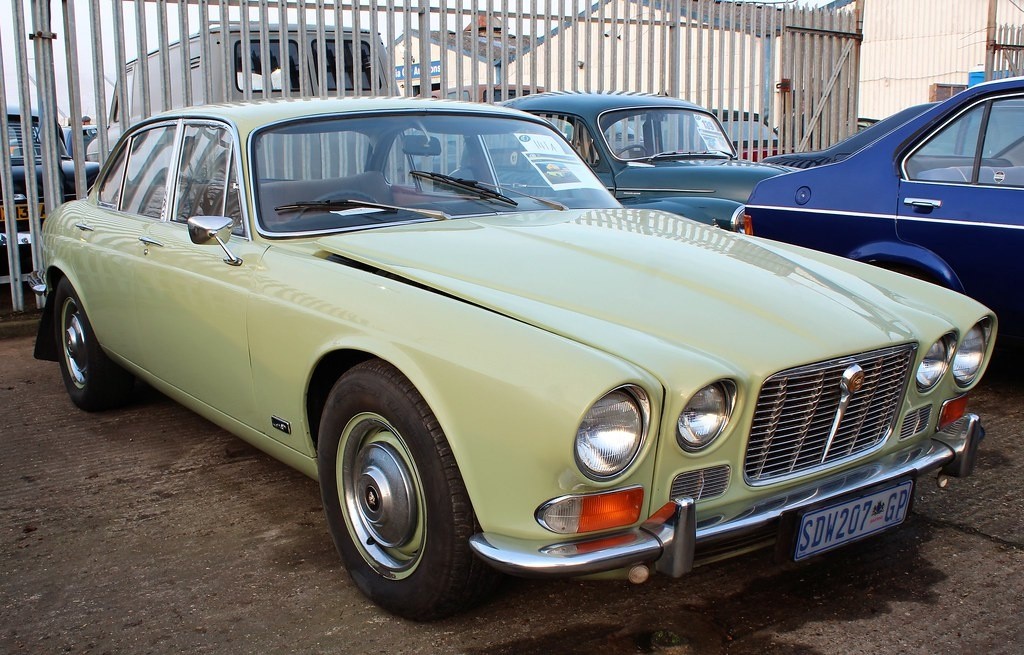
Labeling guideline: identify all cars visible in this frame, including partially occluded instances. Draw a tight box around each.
[0,106,102,288]
[29,94,1001,623]
[410,77,787,164]
[742,74,1024,324]
[502,92,807,234]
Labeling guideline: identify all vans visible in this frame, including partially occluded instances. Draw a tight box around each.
[85,18,402,163]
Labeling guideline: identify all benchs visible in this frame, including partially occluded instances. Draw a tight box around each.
[905,154,1017,184]
[225,171,394,227]
